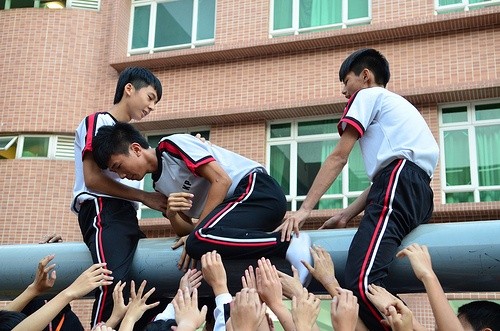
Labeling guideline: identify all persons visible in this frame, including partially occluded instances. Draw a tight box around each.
[69,65,212,331]
[0,233,500,331]
[270,48,440,331]
[91,122,314,290]
